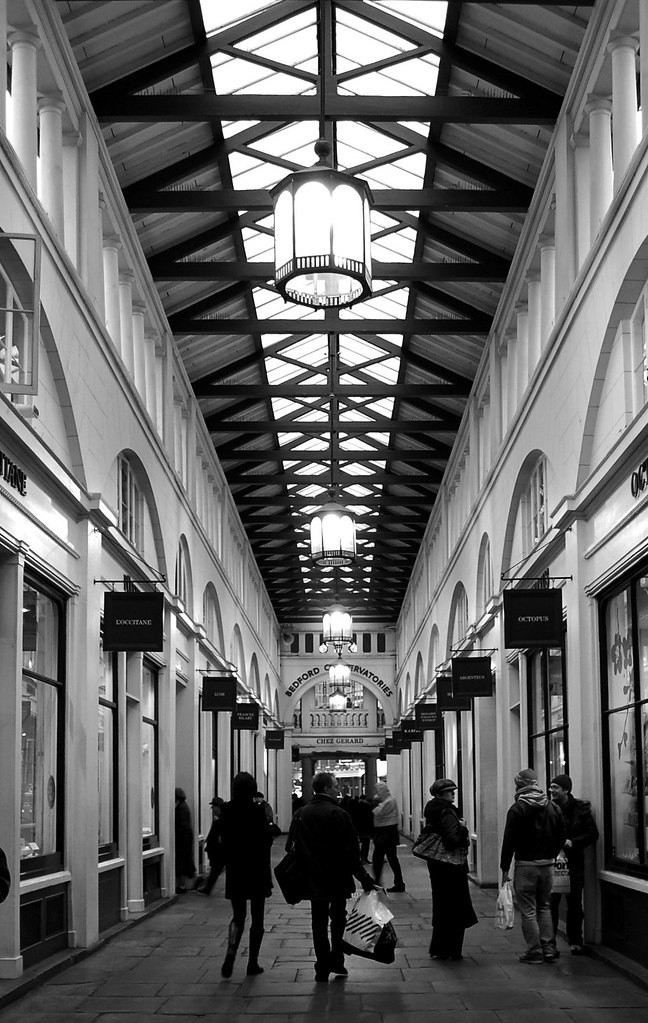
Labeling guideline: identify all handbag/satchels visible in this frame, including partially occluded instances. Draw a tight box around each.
[272,823,281,838]
[337,889,399,964]
[274,852,311,905]
[552,862,571,894]
[411,809,468,867]
[494,881,515,929]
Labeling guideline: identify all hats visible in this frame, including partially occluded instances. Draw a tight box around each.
[514,769,537,787]
[430,779,458,795]
[552,774,571,794]
[255,792,264,799]
[209,797,224,806]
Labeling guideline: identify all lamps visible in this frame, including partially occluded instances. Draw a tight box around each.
[281,633,294,645]
[319,566,357,660]
[307,352,357,568]
[329,687,348,713]
[328,645,351,687]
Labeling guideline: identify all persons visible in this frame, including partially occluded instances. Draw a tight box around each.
[174,788,193,893]
[285,772,378,982]
[371,782,405,893]
[198,797,228,895]
[550,774,599,954]
[291,785,372,865]
[423,778,479,962]
[255,792,273,823]
[221,771,281,978]
[499,768,566,965]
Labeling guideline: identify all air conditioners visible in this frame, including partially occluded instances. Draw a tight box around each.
[268,0,375,309]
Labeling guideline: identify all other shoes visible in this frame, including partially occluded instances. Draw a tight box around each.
[197,889,210,896]
[361,860,371,865]
[247,966,264,975]
[520,950,543,964]
[387,883,405,893]
[315,974,328,982]
[430,954,438,959]
[330,965,348,976]
[222,954,236,978]
[450,956,464,961]
[176,887,187,894]
[543,952,555,963]
[373,881,382,886]
[571,946,584,955]
